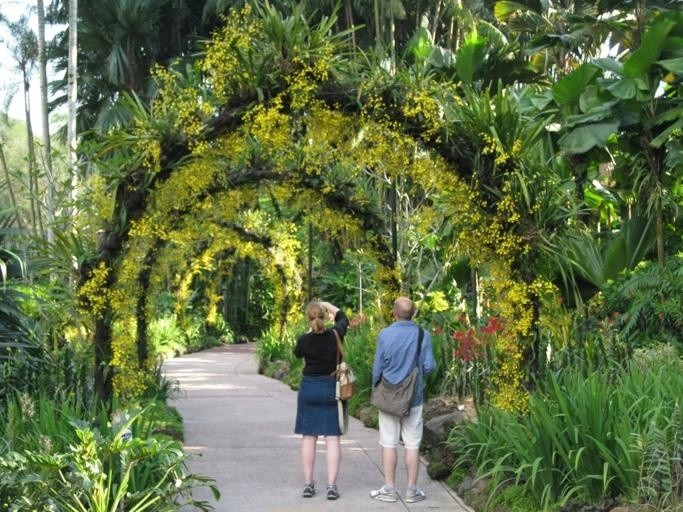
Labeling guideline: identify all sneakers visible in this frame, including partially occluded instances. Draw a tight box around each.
[301,484,316,497]
[371,486,397,502]
[325,485,341,498]
[405,488,426,503]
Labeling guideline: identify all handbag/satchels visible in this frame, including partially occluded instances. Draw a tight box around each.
[335,363,358,400]
[369,367,419,418]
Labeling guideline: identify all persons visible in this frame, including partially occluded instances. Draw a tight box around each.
[293,301,349,500]
[370,297,436,503]
